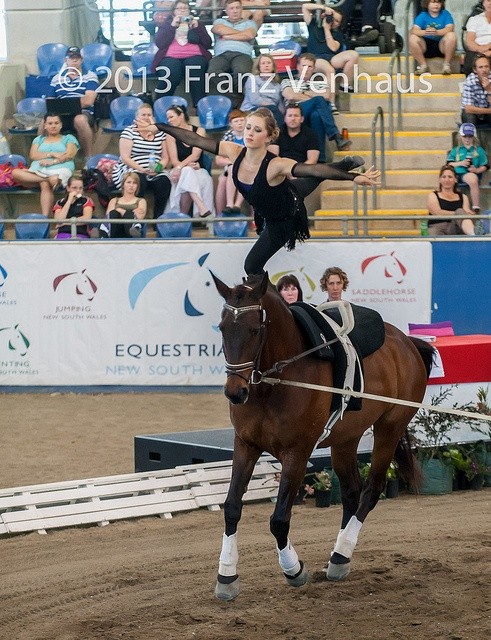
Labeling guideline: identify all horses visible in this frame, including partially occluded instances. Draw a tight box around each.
[207,270,437,602]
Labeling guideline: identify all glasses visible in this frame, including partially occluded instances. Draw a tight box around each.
[174,6,189,10]
[65,55,81,59]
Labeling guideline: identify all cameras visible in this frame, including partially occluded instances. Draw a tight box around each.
[180,15,193,24]
[325,13,334,24]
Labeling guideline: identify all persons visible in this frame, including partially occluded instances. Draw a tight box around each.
[206,0,258,98]
[276,274,302,304]
[11,112,80,216]
[111,102,171,220]
[131,108,383,311]
[52,175,95,238]
[240,0,271,38]
[99,172,147,238]
[151,1,213,107]
[46,46,101,169]
[266,103,320,181]
[319,267,349,302]
[463,0,491,74]
[165,105,216,217]
[446,122,488,212]
[301,3,359,115]
[408,0,457,76]
[460,53,491,127]
[279,53,350,164]
[239,53,285,126]
[215,109,247,215]
[426,164,476,236]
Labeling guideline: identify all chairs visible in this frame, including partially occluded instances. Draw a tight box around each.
[197,95,232,131]
[153,96,187,124]
[481,210,491,233]
[37,43,69,77]
[213,213,248,237]
[156,213,192,237]
[141,223,147,233]
[124,43,159,77]
[103,96,143,133]
[268,40,302,55]
[81,43,112,76]
[8,98,47,133]
[0,154,26,190]
[0,221,5,240]
[86,154,119,170]
[15,214,49,239]
[201,153,212,172]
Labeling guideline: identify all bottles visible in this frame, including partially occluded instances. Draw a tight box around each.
[148,150,155,172]
[342,128,348,150]
[206,107,214,127]
[420,223,427,236]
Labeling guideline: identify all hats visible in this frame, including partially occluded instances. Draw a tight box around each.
[66,45,81,59]
[459,122,477,138]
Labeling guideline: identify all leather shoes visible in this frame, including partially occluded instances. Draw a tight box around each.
[199,210,211,218]
[336,137,352,150]
[232,206,241,214]
[222,207,233,215]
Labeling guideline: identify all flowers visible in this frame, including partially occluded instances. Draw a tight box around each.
[443,446,464,469]
[386,460,398,480]
[262,465,281,492]
[466,458,484,481]
[304,472,332,495]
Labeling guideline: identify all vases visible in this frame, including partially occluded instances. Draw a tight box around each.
[316,491,330,507]
[293,487,304,504]
[388,481,398,497]
[453,471,469,490]
[470,475,485,491]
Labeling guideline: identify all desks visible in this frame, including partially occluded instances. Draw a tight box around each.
[408,334,491,446]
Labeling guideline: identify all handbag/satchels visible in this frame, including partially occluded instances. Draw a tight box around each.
[1,163,30,187]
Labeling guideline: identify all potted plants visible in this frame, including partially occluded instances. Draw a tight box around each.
[407,384,491,496]
[464,387,491,466]
[482,467,491,486]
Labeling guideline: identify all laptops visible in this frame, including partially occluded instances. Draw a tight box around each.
[46,97,82,116]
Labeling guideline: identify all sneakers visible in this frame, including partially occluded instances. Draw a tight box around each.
[330,105,340,115]
[441,63,451,75]
[414,64,430,76]
[338,84,354,93]
[472,206,480,212]
[344,154,366,172]
[51,178,59,190]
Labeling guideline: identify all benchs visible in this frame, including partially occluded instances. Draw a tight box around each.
[139,2,311,42]
